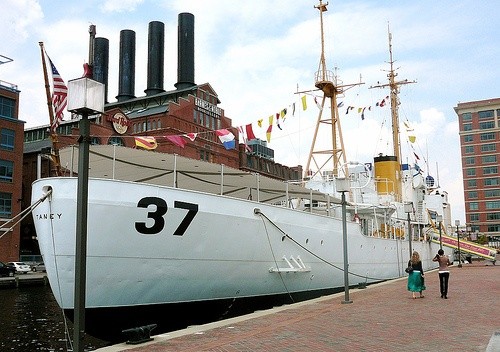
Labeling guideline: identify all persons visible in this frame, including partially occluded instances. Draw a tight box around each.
[407,251,426,298]
[433,249,451,299]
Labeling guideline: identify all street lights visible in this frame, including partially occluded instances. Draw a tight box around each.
[63,78,104,352]
[454,219,463,268]
[475,229,481,262]
[465,223,472,264]
[436,215,443,251]
[334,178,353,304]
[403,203,413,259]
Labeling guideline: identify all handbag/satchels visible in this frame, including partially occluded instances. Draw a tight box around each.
[406,262,413,273]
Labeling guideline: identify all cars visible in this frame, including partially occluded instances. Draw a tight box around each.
[0,262,46,276]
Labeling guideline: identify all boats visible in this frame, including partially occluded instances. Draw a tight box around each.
[31,1,498,342]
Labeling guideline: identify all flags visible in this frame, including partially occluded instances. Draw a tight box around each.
[167,133,197,149]
[337,96,390,120]
[215,119,263,150]
[405,123,420,160]
[50,59,68,128]
[135,137,157,150]
[267,95,323,142]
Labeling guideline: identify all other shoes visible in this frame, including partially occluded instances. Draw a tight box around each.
[445,294,447,299]
[413,296,416,299]
[420,295,424,298]
[441,293,443,298]
